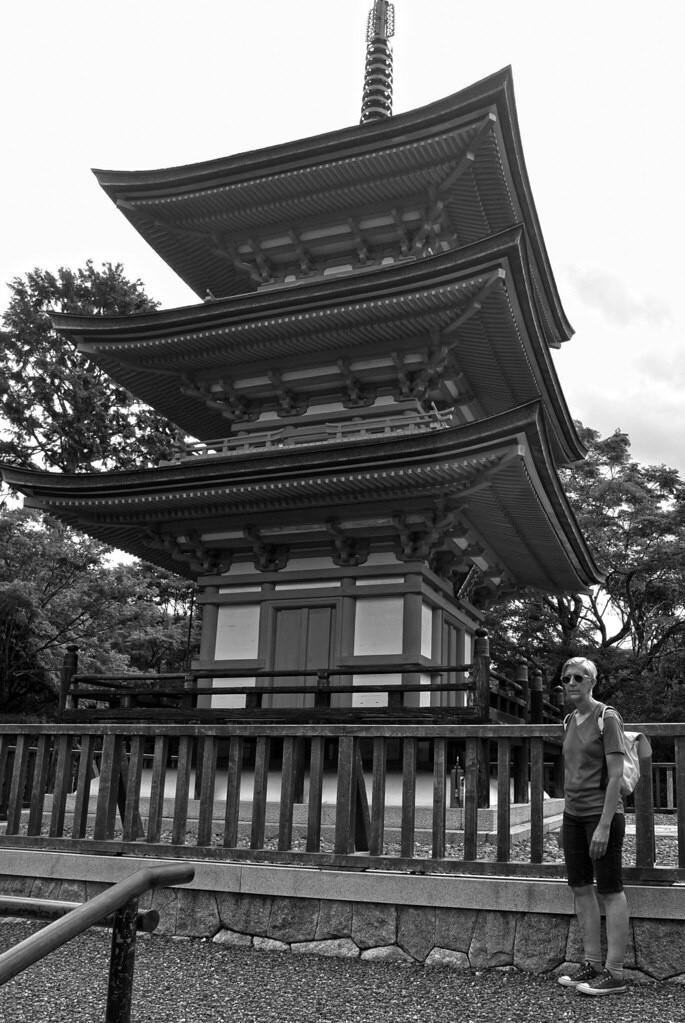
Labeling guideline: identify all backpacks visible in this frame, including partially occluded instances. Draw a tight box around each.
[563,706,652,796]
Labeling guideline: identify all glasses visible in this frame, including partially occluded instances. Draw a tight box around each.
[561,673,591,685]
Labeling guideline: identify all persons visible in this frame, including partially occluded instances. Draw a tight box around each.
[558,657,629,995]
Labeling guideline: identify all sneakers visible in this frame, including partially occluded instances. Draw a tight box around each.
[576,968,626,995]
[558,960,603,987]
